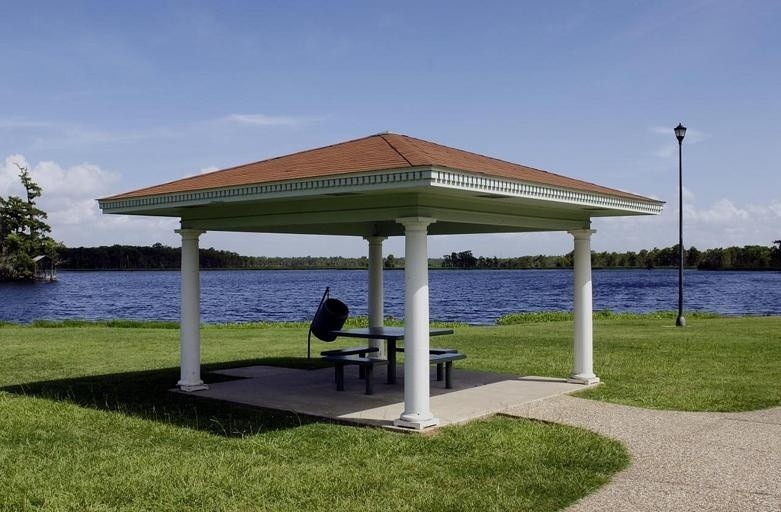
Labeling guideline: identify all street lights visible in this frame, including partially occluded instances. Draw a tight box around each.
[674,122,688,326]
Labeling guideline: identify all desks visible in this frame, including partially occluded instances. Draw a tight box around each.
[332,327,453,384]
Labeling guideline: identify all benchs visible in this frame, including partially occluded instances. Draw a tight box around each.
[429,353,466,389]
[396,347,457,381]
[322,356,387,395]
[321,348,379,384]
